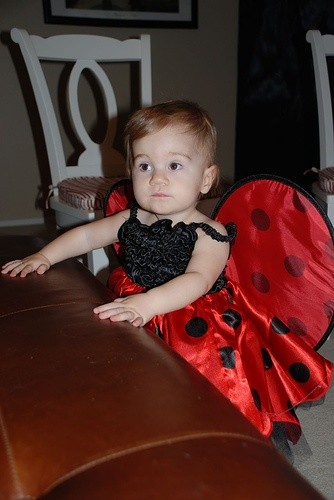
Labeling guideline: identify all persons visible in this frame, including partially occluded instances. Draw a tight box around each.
[1,100,334,470]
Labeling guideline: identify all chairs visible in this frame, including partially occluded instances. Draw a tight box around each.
[306,31,334,228]
[11,27,152,276]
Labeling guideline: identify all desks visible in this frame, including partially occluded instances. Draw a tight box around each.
[0,233,327,500]
